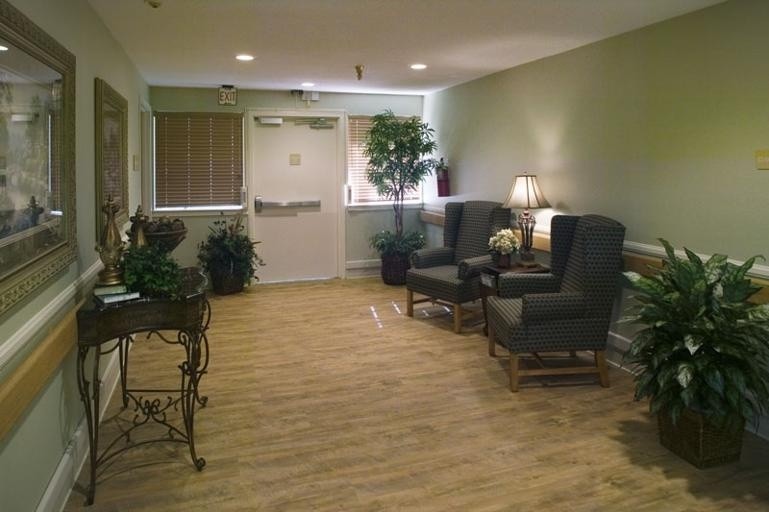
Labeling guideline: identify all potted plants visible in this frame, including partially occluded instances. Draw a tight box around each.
[197,208,266,294]
[363,107,449,285]
[612,237,767,469]
[122,247,192,299]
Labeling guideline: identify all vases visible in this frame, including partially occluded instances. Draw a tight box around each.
[489,253,520,269]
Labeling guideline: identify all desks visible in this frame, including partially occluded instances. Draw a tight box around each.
[76,268,211,506]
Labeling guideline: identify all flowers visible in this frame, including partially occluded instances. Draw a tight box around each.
[486,230,519,253]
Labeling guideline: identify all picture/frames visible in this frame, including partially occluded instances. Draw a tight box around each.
[94,78,130,242]
[0,0,79,325]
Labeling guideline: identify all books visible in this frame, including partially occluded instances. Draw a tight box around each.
[93,284,140,303]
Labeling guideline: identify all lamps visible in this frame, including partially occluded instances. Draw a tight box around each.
[501,172,552,269]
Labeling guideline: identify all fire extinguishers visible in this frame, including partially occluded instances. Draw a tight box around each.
[436,157,451,197]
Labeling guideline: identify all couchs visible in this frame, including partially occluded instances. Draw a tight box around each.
[485,215,625,393]
[406,200,511,334]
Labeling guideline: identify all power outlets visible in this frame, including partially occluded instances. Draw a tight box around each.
[755,150,769,170]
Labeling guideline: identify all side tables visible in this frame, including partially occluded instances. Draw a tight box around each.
[480,263,550,336]
